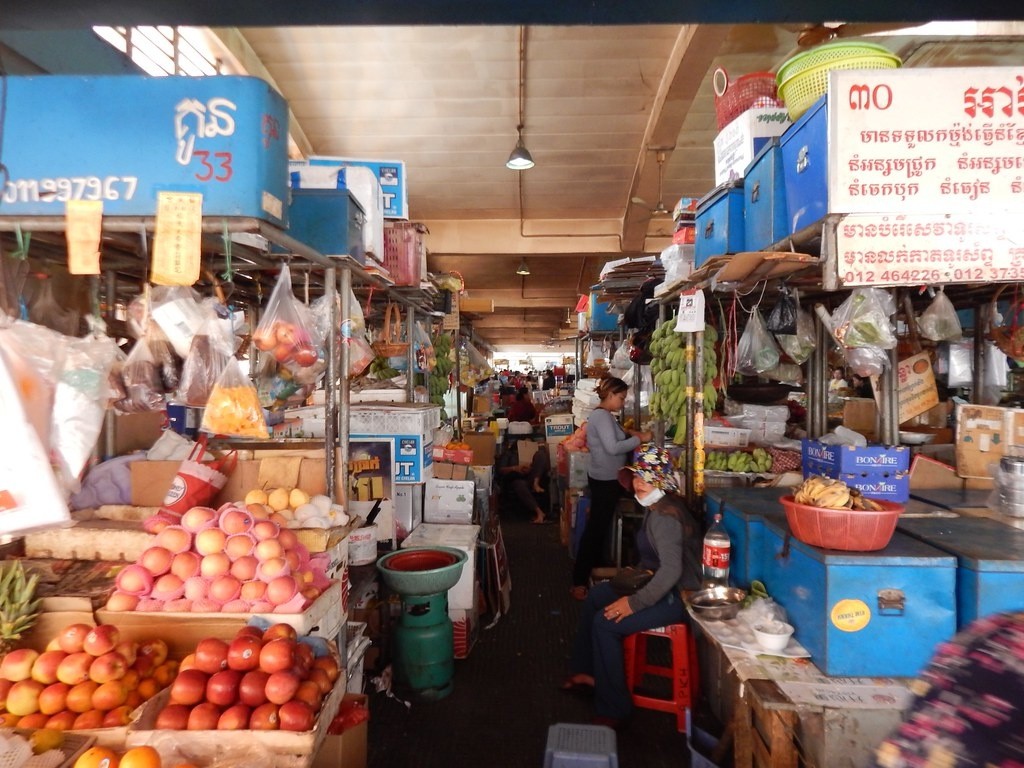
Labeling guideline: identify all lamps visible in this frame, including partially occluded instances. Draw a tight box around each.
[506,125,535,170]
[516,257,531,274]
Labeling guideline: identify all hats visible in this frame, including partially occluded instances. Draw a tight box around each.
[616,442,680,497]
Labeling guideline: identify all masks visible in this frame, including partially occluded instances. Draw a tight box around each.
[634,489,666,508]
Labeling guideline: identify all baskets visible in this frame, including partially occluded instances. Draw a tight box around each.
[369,303,409,357]
[778,494,905,552]
[712,41,902,133]
[987,283,1024,361]
[376,546,468,594]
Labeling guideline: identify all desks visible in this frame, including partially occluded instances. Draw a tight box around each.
[347,553,391,671]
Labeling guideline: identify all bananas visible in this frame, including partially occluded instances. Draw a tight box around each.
[649,313,718,424]
[677,446,774,475]
[370,358,400,380]
[416,333,453,420]
[793,472,886,512]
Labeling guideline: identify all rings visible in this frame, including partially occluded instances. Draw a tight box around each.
[616,611,620,615]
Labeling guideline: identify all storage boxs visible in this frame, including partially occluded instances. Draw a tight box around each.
[0,388,593,768]
[576,292,629,331]
[0,74,427,288]
[671,93,830,269]
[702,400,1024,678]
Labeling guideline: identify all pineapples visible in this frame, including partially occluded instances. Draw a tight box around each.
[0,560,45,660]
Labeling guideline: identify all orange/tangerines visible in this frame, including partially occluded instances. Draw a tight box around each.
[72,745,163,768]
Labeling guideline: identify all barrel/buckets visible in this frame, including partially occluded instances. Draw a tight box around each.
[348,520,379,567]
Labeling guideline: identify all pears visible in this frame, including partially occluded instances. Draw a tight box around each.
[244,480,342,530]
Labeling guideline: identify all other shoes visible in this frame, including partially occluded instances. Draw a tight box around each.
[570,585,590,602]
[559,674,596,693]
[590,567,620,580]
[585,715,622,730]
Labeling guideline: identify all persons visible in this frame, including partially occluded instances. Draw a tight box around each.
[559,442,701,729]
[498,368,556,423]
[827,364,875,399]
[497,436,549,523]
[569,378,654,601]
[873,607,1024,768]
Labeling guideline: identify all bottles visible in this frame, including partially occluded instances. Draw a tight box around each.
[700,513,731,590]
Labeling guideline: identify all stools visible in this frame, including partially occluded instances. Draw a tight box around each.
[622,620,701,733]
[543,723,618,768]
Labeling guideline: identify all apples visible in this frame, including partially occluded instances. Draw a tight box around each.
[1,624,181,755]
[154,623,341,732]
[104,503,325,614]
[254,321,319,367]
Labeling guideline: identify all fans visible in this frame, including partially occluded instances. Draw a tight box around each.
[630,151,675,225]
[766,21,928,72]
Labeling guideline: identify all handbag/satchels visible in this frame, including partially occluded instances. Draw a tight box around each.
[732,284,964,375]
[26,261,438,441]
[157,433,238,525]
[617,564,654,590]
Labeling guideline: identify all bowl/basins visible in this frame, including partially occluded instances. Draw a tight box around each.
[899,430,937,445]
[750,619,795,649]
[728,384,791,401]
[376,545,469,595]
[687,587,746,619]
